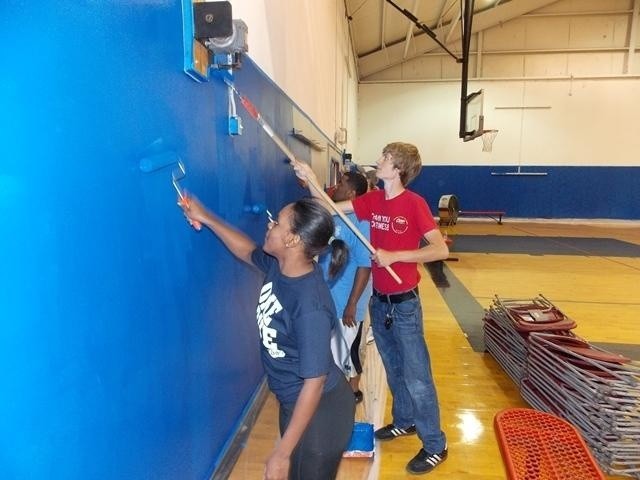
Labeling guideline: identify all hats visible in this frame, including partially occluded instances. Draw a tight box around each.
[354,163,381,187]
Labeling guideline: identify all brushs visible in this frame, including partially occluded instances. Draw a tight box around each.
[139,151,203,230]
[291,127,325,151]
[252,201,274,222]
[211,67,259,122]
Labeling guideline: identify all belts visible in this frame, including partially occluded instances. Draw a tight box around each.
[371,285,419,305]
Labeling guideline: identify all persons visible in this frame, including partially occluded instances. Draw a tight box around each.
[184,194,356,480]
[289,142,451,474]
[298,166,379,405]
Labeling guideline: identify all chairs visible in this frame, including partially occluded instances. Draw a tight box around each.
[482,295,640,480]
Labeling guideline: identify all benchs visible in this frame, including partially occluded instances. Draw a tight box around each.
[459,211,505,225]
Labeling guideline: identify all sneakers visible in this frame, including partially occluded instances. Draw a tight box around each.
[374,421,417,442]
[404,441,449,476]
[352,388,365,405]
